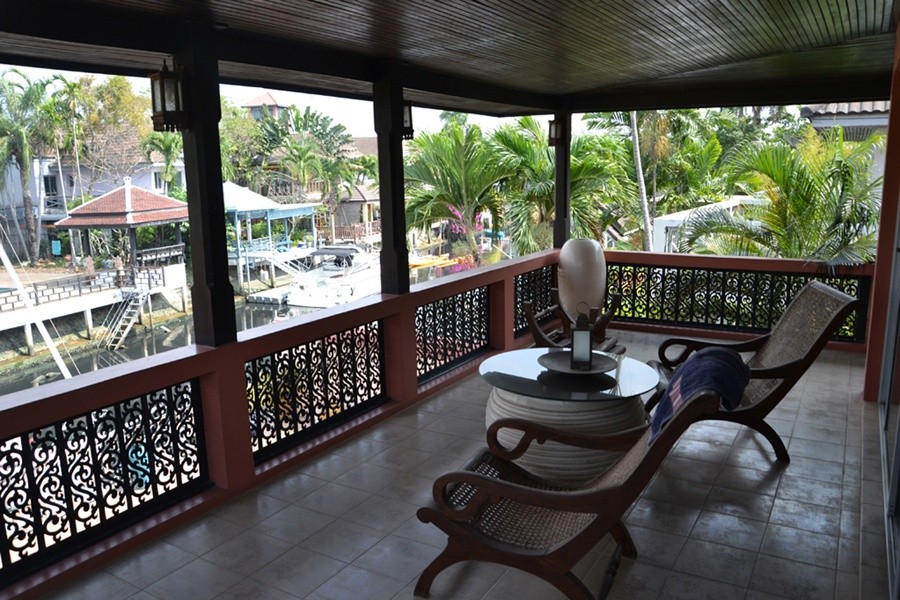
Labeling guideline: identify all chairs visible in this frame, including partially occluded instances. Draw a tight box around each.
[523,238,627,357]
[413,346,750,600]
[641,280,861,464]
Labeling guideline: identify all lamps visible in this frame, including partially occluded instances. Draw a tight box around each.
[404,100,414,140]
[571,302,593,370]
[151,58,188,132]
[548,114,563,146]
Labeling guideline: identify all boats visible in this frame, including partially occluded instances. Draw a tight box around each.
[408,252,459,270]
[30,371,61,386]
[285,243,381,308]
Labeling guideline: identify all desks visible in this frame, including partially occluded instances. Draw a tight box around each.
[478,349,660,490]
